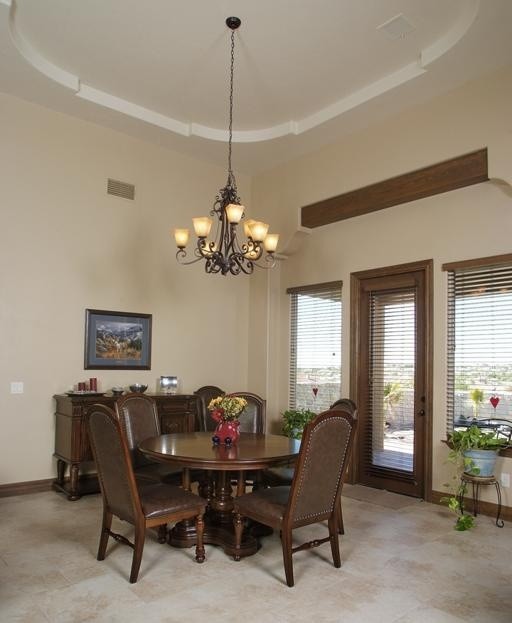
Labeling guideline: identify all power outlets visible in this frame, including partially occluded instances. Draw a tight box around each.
[10,383,24,394]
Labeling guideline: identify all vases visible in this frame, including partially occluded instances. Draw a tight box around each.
[214,421,241,445]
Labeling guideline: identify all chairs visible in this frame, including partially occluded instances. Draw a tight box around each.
[224,392,266,487]
[193,385,226,432]
[115,392,198,483]
[232,409,358,587]
[83,403,209,584]
[268,399,359,535]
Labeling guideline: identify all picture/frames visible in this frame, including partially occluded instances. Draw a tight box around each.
[83,309,152,370]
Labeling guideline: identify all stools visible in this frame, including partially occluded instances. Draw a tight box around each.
[459,474,505,528]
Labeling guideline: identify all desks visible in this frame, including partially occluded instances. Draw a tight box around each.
[136,433,301,556]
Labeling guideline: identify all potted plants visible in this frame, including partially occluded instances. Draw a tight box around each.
[280,408,318,440]
[440,424,512,531]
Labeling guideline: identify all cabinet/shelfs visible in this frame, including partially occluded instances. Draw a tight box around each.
[52,394,199,500]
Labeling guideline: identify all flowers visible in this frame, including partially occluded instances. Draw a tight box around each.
[207,396,247,422]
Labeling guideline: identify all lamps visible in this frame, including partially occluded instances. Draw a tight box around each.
[174,16,280,277]
